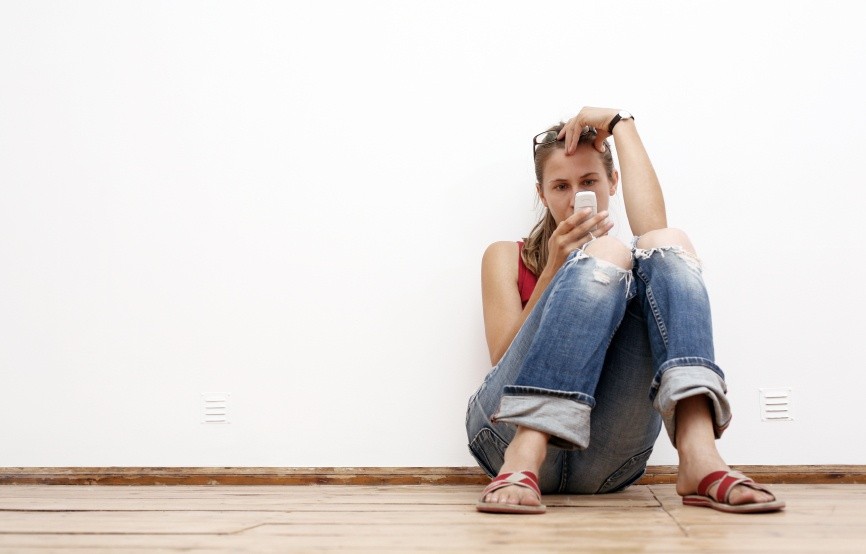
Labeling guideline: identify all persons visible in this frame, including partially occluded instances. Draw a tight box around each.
[466,105,786,512]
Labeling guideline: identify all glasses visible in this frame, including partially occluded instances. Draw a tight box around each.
[533,129,612,159]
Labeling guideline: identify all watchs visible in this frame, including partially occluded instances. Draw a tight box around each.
[607,110,635,134]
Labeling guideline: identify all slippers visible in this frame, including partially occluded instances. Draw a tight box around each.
[477,470,546,514]
[683,471,785,512]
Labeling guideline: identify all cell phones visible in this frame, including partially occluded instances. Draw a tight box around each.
[574,192,598,240]
